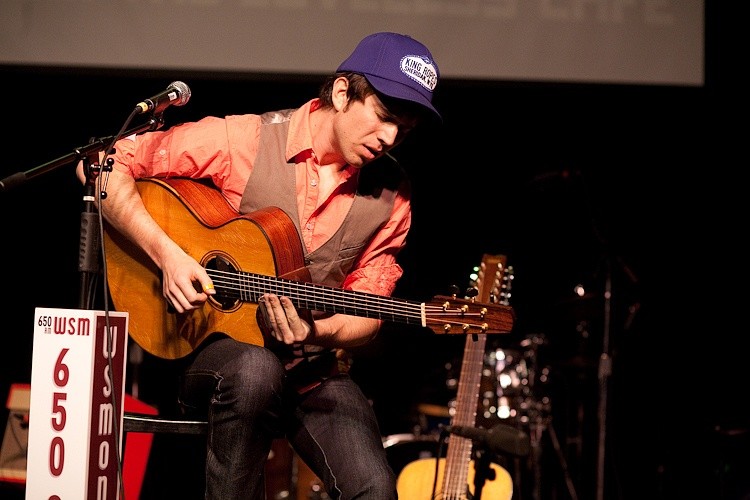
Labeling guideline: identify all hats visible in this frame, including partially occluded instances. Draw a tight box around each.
[335,32,439,116]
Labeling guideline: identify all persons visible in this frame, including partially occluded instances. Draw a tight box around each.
[75,32,441,500]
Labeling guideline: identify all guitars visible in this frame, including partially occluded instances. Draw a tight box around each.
[99,177,518,361]
[395,255,515,500]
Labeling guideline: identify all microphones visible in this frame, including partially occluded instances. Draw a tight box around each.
[534,169,582,199]
[448,421,530,459]
[136,80,192,114]
